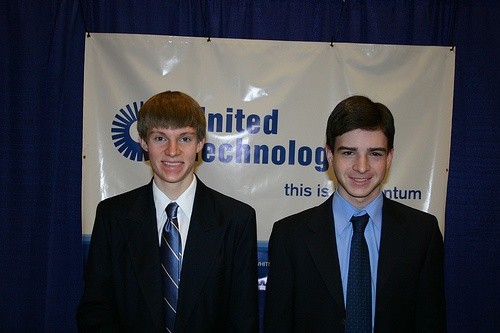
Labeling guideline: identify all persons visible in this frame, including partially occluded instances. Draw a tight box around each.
[74,89,257,333]
[259,94,447,333]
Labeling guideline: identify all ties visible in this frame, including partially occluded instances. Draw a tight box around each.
[160,201,183,332]
[345,214,374,332]
